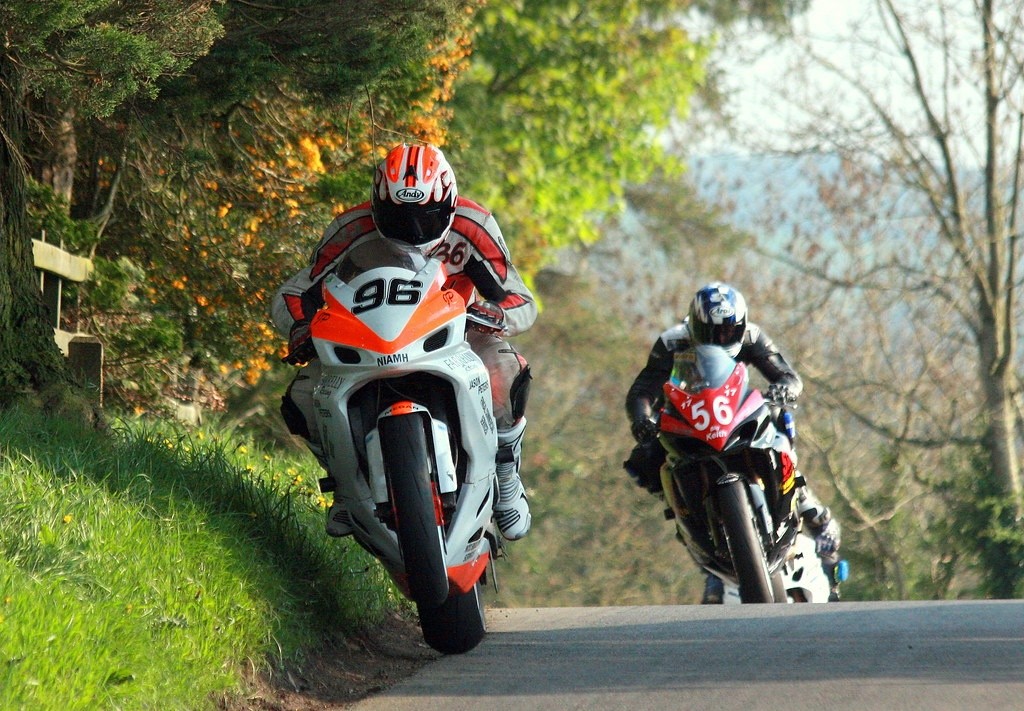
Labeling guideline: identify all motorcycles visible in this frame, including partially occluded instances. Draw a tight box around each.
[279,240,515,657]
[633,343,849,605]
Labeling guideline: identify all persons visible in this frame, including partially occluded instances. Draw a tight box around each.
[619,280,848,605]
[270,140,539,540]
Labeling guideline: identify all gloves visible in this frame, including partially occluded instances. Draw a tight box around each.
[290,319,319,365]
[768,379,799,405]
[632,418,658,445]
[465,299,508,335]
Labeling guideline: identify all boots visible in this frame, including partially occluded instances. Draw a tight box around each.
[304,436,355,537]
[798,493,841,557]
[492,416,532,540]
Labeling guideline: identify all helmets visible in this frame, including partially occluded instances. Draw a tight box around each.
[369,140,459,257]
[688,280,749,360]
